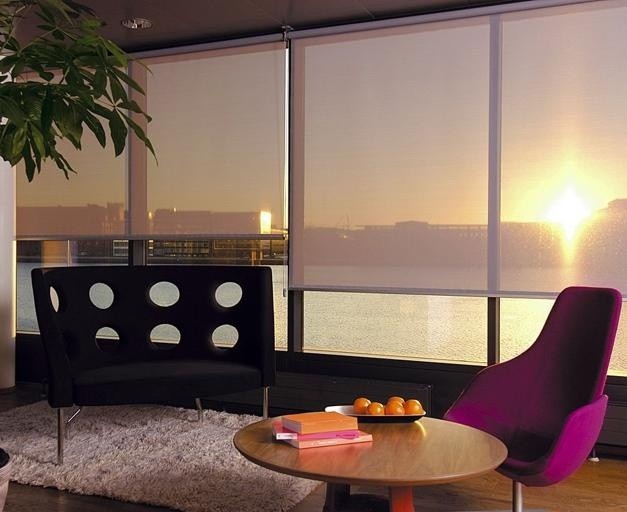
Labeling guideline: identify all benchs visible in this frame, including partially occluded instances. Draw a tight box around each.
[30,266,275,465]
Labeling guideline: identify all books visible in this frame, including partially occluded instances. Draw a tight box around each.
[271,411,373,449]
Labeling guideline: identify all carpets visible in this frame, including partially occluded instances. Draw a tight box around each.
[0,399,322,510]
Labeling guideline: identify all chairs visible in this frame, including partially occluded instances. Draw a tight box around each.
[443,286,622,512]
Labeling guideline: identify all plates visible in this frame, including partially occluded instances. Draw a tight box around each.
[324,404,426,422]
